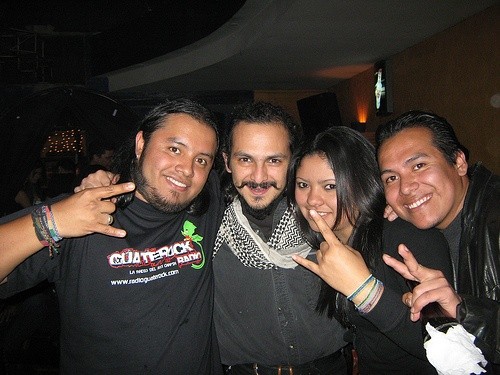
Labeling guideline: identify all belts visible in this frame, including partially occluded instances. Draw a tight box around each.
[239,343,353,375]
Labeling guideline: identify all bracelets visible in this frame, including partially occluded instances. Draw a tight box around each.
[345,274,384,315]
[31,203,63,257]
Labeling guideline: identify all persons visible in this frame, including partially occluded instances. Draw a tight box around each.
[375,109,500,375]
[74,98,398,375]
[284,124,458,375]
[72,140,116,191]
[0,154,48,213]
[0,97,237,375]
